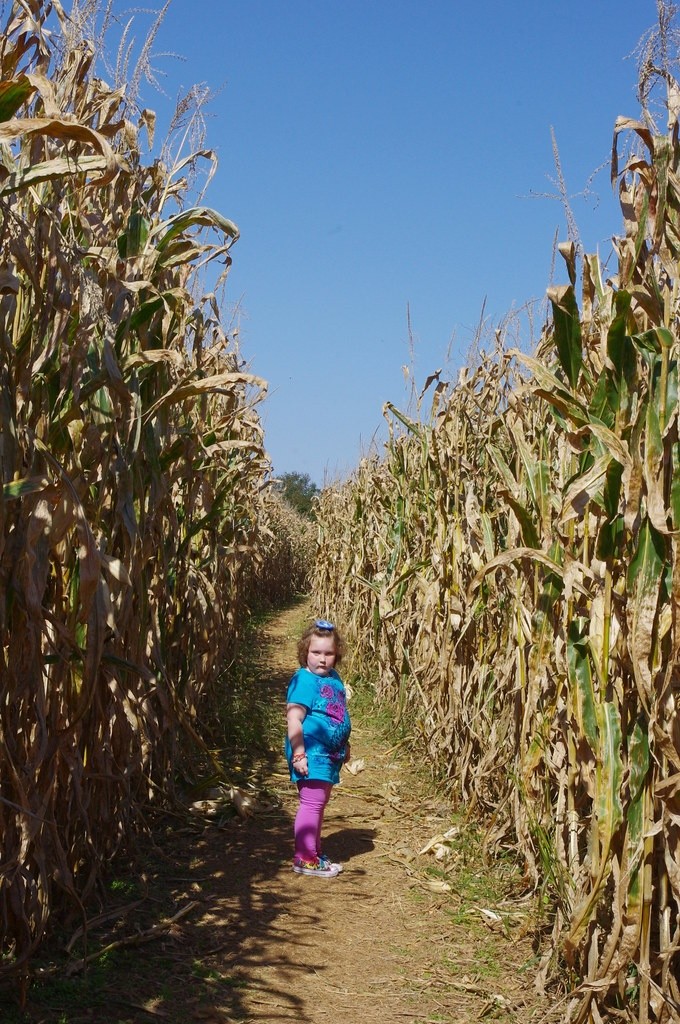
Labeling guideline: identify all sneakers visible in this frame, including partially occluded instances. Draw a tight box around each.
[292,855,338,878]
[316,852,343,873]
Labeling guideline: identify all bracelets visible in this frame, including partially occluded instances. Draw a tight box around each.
[291,752,306,763]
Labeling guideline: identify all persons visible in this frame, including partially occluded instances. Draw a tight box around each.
[283,621,352,879]
